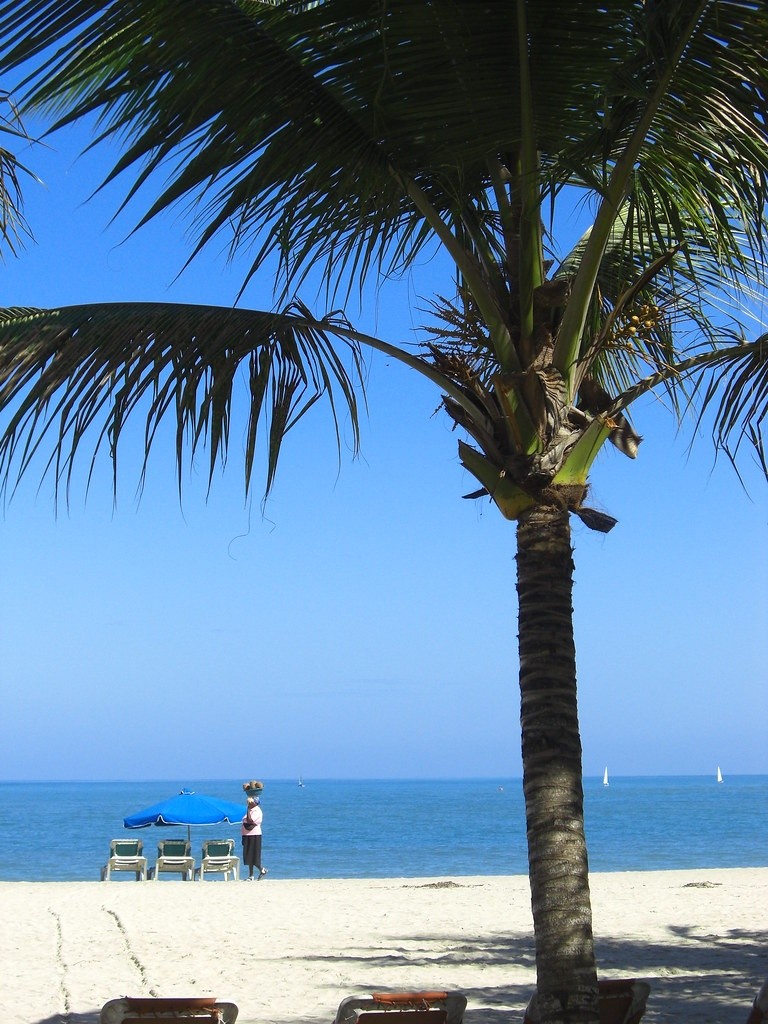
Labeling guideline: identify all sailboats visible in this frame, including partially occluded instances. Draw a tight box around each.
[602,766,609,786]
[716,766,725,784]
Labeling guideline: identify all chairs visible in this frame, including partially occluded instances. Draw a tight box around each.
[147,839,195,881]
[99,998,239,1024]
[524,978,651,1024]
[332,992,467,1024]
[194,838,239,881]
[101,838,148,881]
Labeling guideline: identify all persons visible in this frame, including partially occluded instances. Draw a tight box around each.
[241,796,269,881]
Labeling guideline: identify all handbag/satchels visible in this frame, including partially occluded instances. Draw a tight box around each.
[243,823,257,830]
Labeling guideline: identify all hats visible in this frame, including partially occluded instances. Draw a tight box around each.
[252,796,260,805]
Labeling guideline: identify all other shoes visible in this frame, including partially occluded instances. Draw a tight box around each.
[257,868,268,881]
[244,876,254,881]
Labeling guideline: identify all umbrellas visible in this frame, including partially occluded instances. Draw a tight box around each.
[122,787,249,830]
